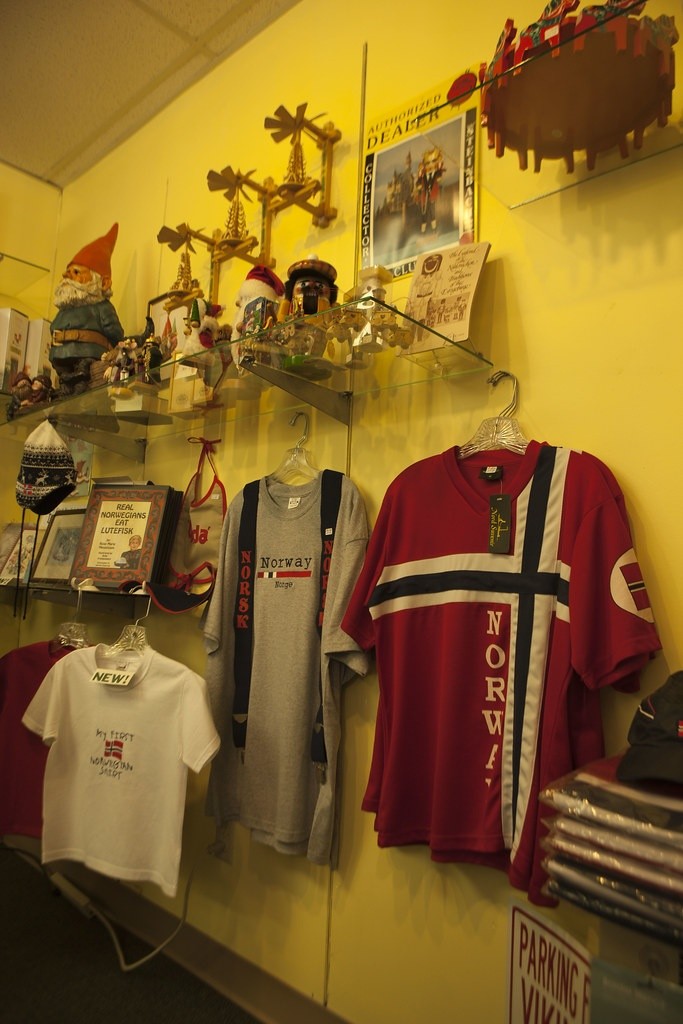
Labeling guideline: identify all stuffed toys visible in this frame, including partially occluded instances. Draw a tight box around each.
[182,265,284,387]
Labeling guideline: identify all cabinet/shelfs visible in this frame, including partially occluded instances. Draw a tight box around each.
[1,297,495,615]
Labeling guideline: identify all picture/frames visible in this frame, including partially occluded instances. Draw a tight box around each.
[28,507,88,583]
[0,528,46,585]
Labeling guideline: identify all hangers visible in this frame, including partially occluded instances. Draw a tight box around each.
[266,412,321,485]
[50,586,90,654]
[96,585,151,661]
[455,371,529,459]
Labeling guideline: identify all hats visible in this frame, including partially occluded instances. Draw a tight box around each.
[614,670,683,781]
[15,419,76,515]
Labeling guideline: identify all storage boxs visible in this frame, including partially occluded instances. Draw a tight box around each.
[22,318,57,388]
[0,306,30,393]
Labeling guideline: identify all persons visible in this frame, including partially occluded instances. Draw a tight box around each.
[119,536,142,570]
[3,222,121,421]
